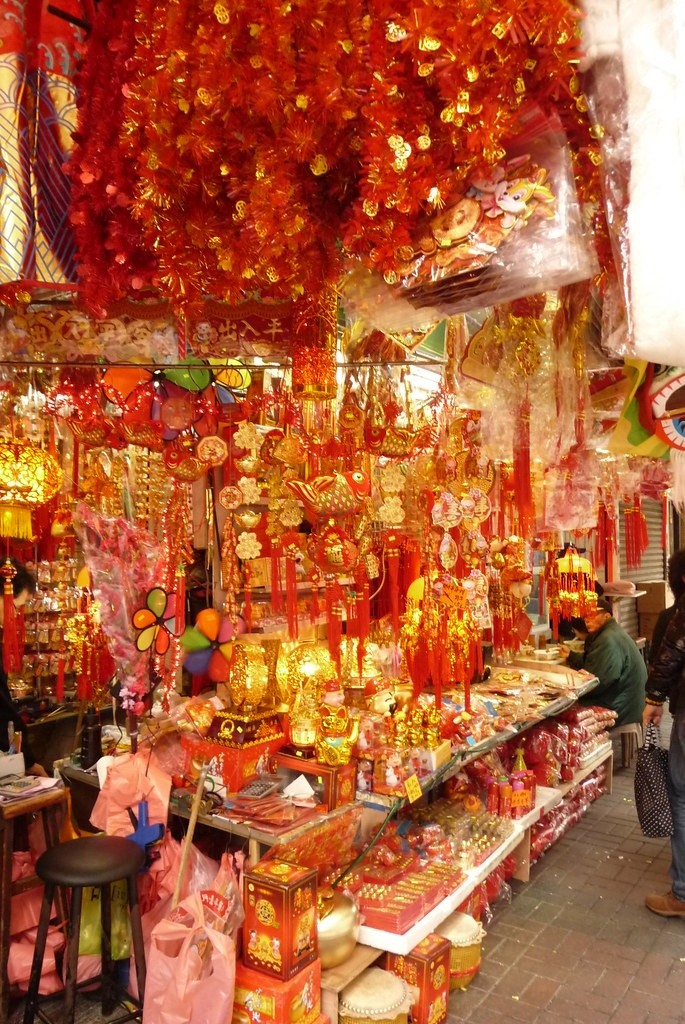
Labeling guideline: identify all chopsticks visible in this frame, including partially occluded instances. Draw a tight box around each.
[570,637,579,644]
[548,647,562,651]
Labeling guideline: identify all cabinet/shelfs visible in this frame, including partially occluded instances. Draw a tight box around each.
[61,660,620,1022]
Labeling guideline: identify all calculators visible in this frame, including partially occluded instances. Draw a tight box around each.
[237,775,284,800]
[0,774,41,794]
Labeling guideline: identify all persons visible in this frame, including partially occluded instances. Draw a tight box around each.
[0,558,52,852]
[548,542,613,645]
[555,597,648,732]
[634,547,685,920]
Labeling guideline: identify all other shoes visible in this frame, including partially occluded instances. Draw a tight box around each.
[645,890,685,919]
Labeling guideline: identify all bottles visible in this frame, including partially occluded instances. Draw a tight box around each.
[80,705,101,769]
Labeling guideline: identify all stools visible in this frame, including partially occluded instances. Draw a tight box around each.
[0,784,147,1023]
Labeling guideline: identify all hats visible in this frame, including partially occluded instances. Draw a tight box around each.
[557,542,586,553]
[596,599,613,616]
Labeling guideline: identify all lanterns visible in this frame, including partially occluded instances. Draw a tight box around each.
[0,435,64,543]
[291,280,338,401]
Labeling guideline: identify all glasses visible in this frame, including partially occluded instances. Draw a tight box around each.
[13,600,22,616]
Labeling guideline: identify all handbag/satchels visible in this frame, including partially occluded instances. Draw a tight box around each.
[634,721,675,838]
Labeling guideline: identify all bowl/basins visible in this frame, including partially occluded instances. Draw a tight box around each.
[563,641,586,651]
[534,650,559,660]
